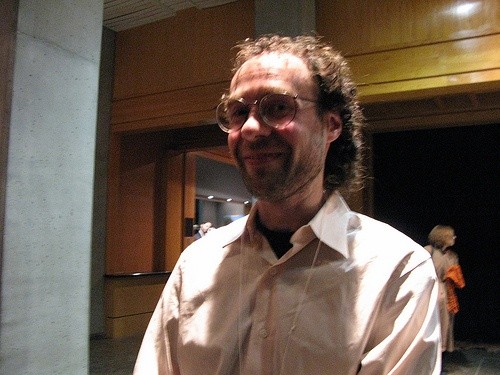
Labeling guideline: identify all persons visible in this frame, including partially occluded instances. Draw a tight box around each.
[132,33,445,375]
[193,220,216,241]
[421,225,465,375]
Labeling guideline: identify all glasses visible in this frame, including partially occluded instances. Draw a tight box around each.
[215,93,330,134]
[448,234,457,240]
